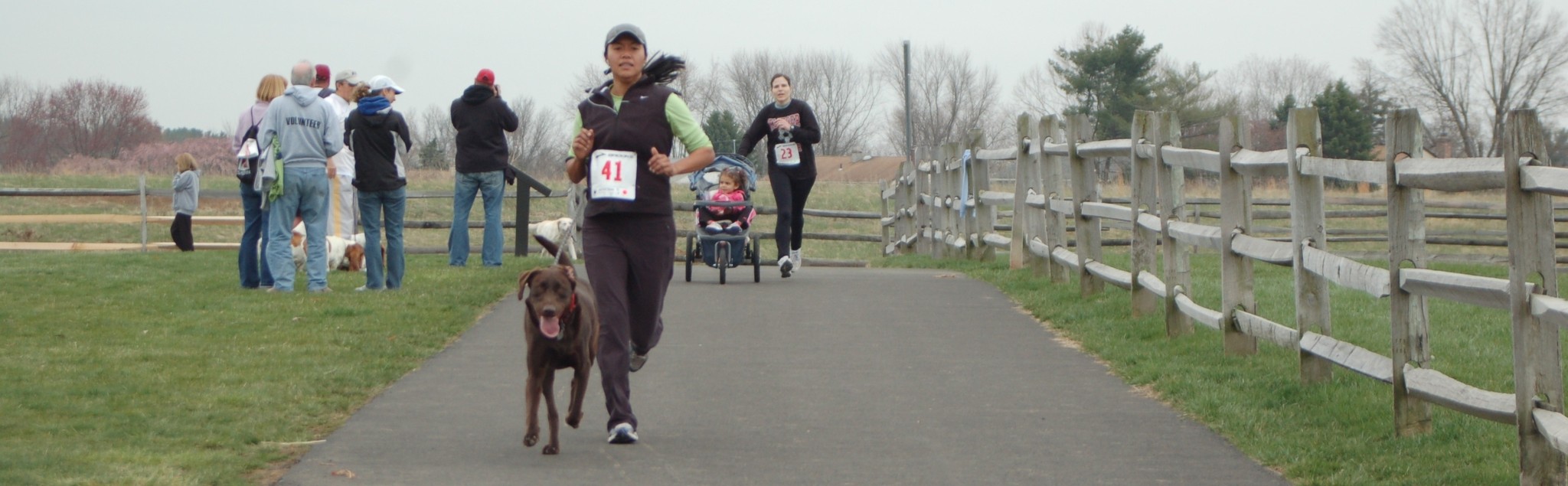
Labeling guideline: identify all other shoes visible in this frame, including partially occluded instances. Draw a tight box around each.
[355,284,388,292]
[319,287,334,292]
[726,222,743,235]
[705,222,722,235]
[629,345,647,372]
[777,255,793,278]
[267,286,292,292]
[608,422,638,444]
[790,247,801,272]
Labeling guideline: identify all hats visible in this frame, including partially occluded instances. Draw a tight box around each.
[604,24,647,75]
[336,70,363,84]
[476,69,494,85]
[368,76,404,95]
[315,64,330,79]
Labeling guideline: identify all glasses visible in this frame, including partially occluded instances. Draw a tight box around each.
[342,82,357,86]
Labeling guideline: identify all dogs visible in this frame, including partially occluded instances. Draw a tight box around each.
[518,265,598,455]
[289,229,386,273]
[529,217,578,262]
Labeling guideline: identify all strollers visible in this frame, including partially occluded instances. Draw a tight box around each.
[684,154,762,284]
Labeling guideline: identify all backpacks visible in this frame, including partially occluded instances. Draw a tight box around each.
[236,106,264,184]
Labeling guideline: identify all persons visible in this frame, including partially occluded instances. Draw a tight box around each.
[234,61,413,293]
[565,25,716,442]
[170,152,200,253]
[697,167,756,233]
[449,69,519,267]
[736,74,822,277]
